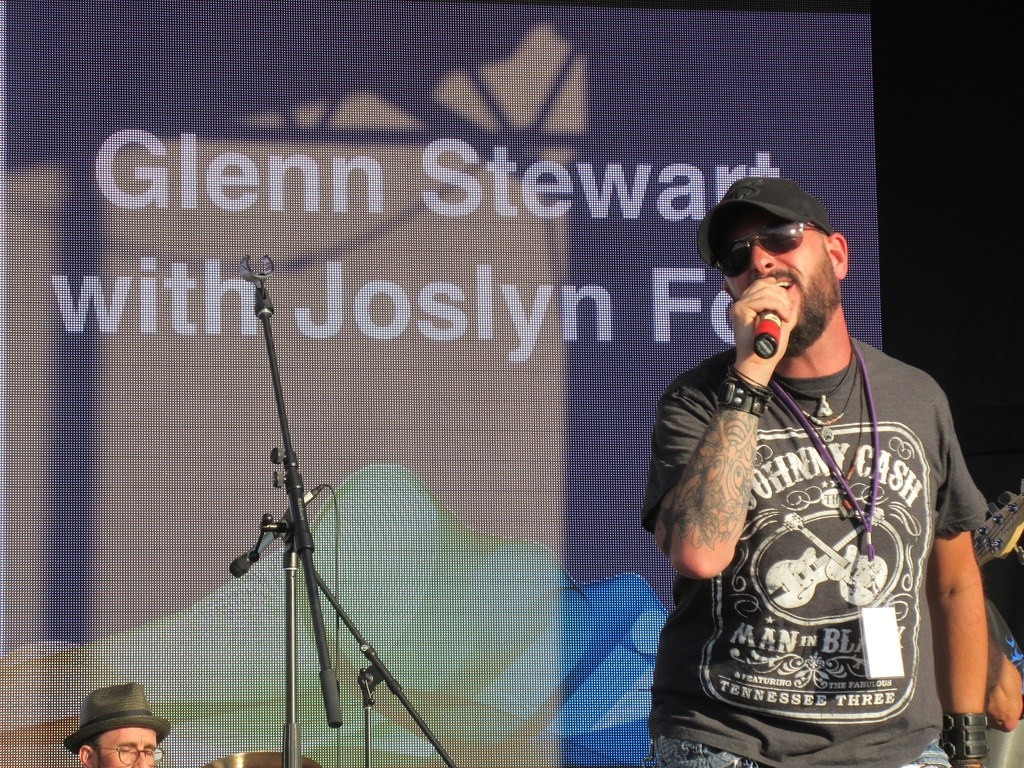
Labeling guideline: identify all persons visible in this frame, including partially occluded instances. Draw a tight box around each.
[63,684,171,768]
[641,178,990,768]
[981,591,1024,731]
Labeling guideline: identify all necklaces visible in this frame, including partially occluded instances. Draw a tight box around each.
[777,347,865,518]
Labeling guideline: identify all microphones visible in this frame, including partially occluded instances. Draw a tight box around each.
[229,484,324,577]
[755,309,782,358]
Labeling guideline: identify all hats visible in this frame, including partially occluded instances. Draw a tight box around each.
[695,176,833,264]
[63,682,170,754]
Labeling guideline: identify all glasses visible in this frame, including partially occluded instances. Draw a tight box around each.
[709,223,828,278]
[95,742,163,765]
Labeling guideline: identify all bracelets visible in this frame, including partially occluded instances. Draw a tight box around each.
[718,367,774,416]
